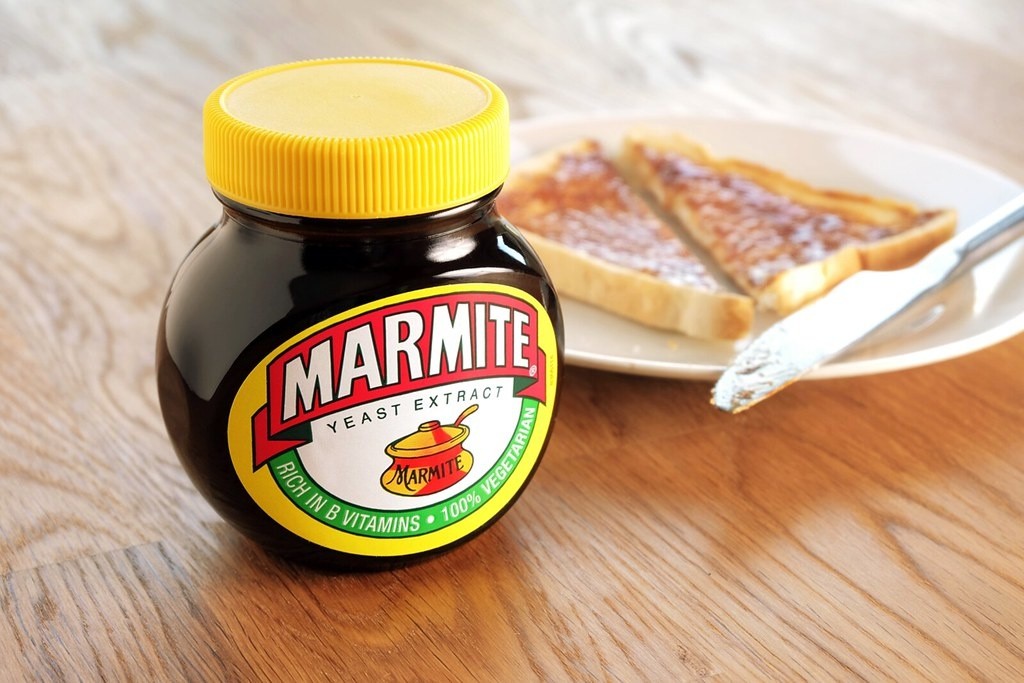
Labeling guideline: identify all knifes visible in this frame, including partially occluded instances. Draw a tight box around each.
[710,193,1024,415]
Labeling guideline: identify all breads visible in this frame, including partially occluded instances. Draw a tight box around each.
[492,140,754,345]
[629,128,956,314]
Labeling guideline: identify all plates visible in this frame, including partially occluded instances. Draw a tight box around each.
[497,108,1024,383]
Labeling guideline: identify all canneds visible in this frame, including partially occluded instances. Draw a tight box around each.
[153,59,565,575]
